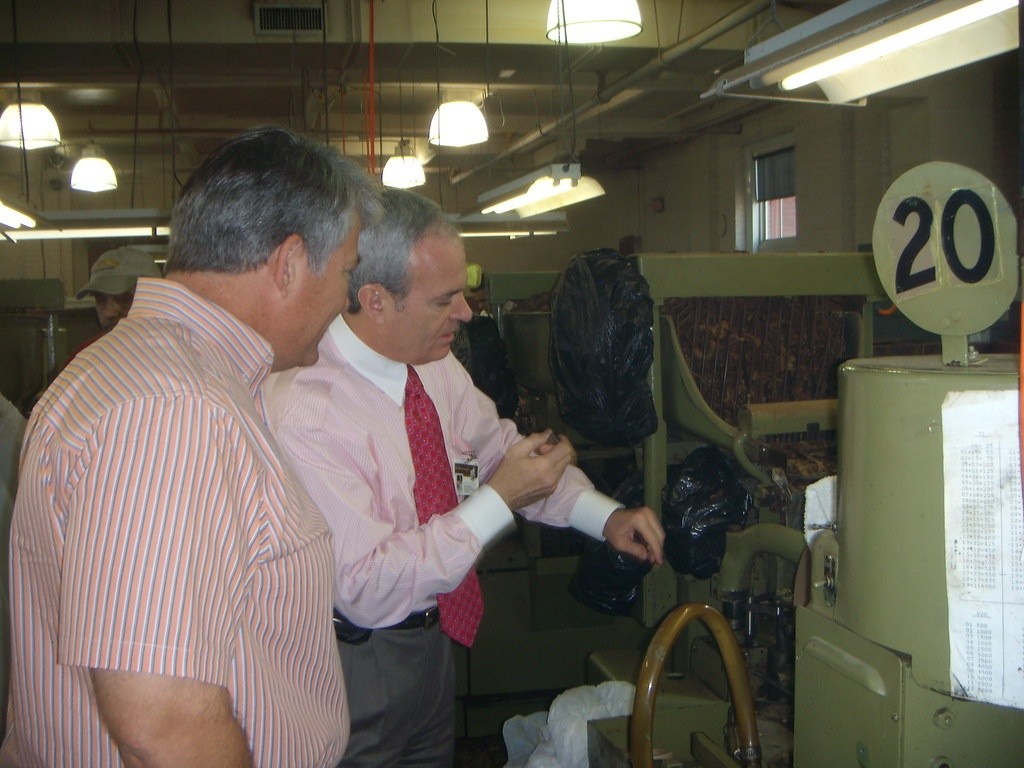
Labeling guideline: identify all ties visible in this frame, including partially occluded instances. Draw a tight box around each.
[404,364,485,648]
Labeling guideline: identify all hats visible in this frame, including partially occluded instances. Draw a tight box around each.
[76,246,163,299]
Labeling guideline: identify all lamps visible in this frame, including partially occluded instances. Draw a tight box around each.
[0,1,649,193]
[457,163,605,219]
[448,210,572,239]
[0,208,169,245]
[0,197,64,232]
[699,0,1020,108]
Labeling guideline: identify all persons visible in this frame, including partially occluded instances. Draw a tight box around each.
[262,187,664,768]
[457,476,462,490]
[0,126,384,768]
[33,248,163,398]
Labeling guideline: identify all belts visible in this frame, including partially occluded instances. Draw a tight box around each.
[382,606,441,630]
